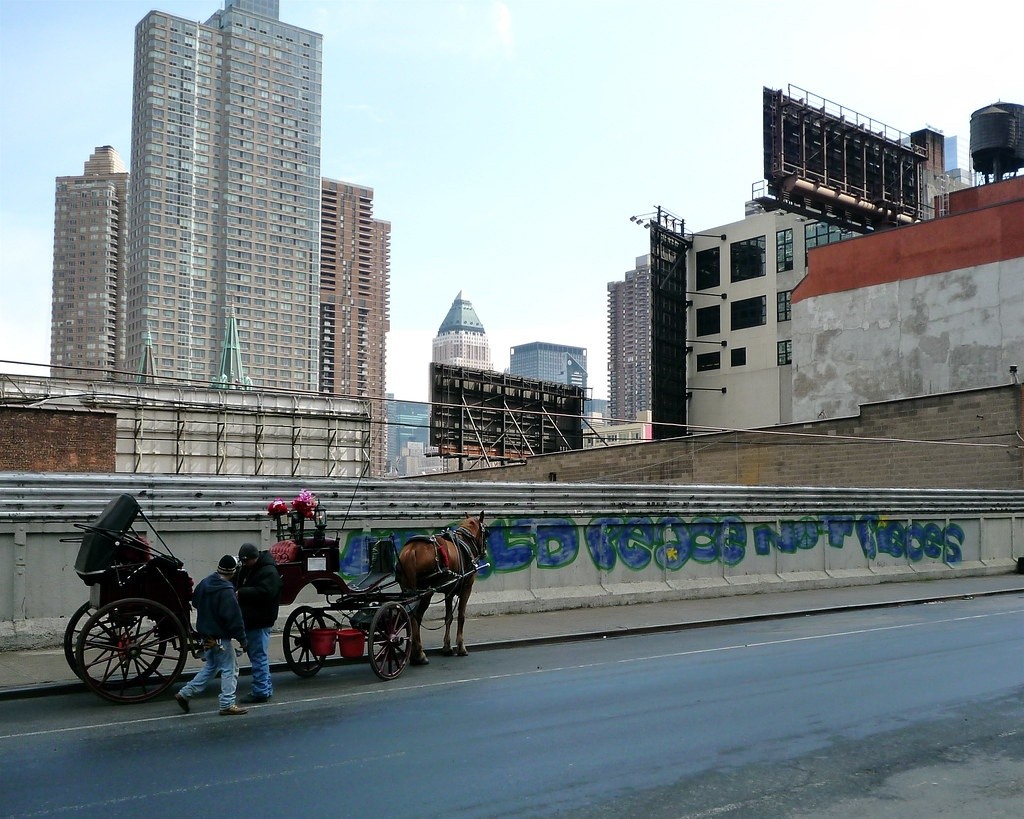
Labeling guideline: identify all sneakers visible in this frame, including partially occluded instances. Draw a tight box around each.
[220,705,249,715]
[175,694,190,713]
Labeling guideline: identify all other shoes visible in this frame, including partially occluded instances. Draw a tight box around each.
[240,692,272,704]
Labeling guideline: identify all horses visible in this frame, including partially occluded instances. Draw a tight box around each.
[394,510,490,666]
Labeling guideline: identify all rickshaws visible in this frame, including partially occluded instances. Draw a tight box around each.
[52,493,251,702]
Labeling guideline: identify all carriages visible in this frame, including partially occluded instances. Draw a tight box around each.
[262,488,491,681]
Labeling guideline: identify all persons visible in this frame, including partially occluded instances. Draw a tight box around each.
[229,542,279,702]
[174,555,249,715]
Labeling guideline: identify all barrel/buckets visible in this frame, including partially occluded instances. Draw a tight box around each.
[336,630,365,658]
[312,629,337,655]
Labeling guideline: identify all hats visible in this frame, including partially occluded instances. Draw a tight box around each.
[217,555,236,575]
[239,543,259,561]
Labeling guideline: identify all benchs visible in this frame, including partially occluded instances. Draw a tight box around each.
[266,537,299,562]
[298,533,338,548]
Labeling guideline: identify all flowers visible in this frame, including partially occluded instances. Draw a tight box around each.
[291,488,318,518]
[266,497,288,517]
